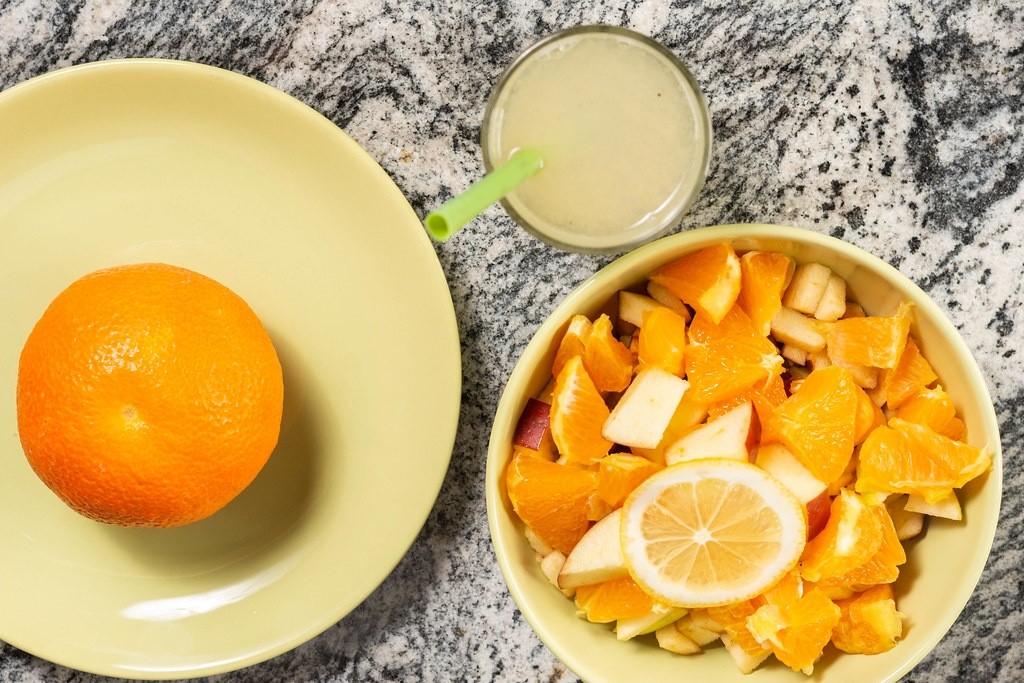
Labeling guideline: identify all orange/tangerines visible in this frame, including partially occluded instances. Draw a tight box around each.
[506,245,993,676]
[16,264,284,526]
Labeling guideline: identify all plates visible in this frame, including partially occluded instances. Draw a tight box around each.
[0,59,462,681]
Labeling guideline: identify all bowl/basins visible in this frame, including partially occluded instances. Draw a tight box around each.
[486,222,1002,682]
[481,26,713,254]
